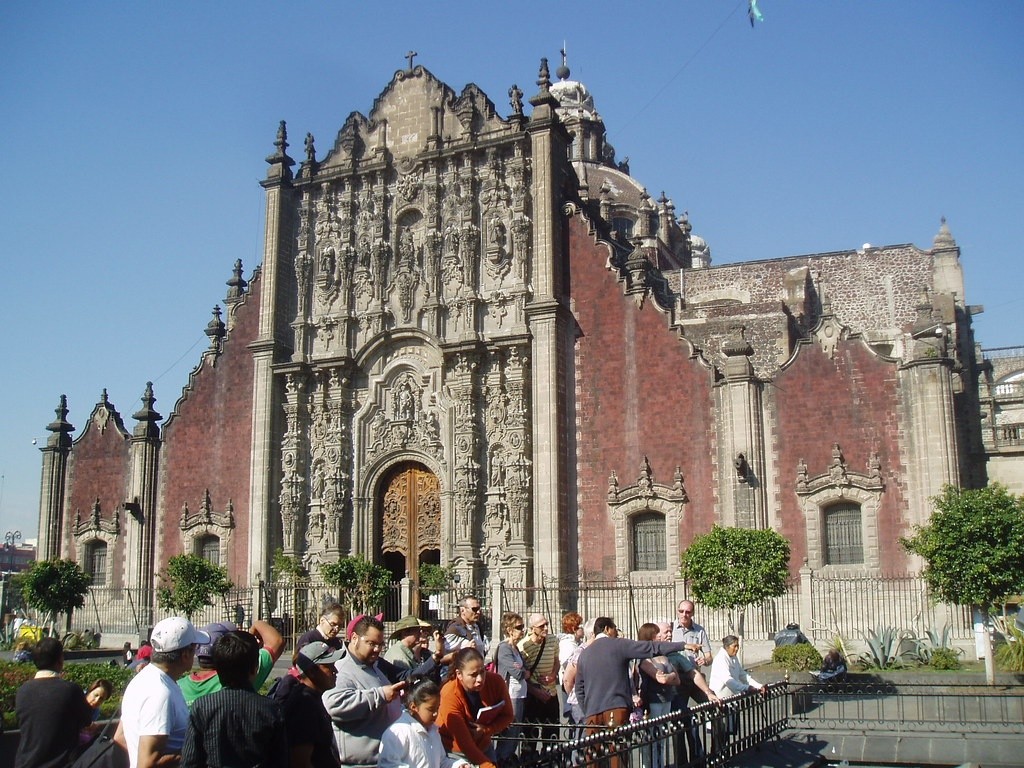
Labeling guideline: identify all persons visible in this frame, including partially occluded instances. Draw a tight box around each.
[774,622,807,646]
[15,595,766,768]
[490,212,504,244]
[399,226,414,248]
[491,451,503,487]
[399,386,411,418]
[314,464,324,498]
[323,242,333,271]
[357,236,370,267]
[822,648,847,682]
[444,221,458,252]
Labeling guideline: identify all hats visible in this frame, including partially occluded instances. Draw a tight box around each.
[347,613,383,642]
[389,617,432,640]
[194,621,238,658]
[295,641,347,674]
[150,617,210,653]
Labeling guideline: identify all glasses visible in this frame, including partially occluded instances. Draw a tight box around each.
[534,621,550,630]
[309,647,337,667]
[679,609,694,614]
[323,616,343,631]
[508,624,526,631]
[462,607,481,613]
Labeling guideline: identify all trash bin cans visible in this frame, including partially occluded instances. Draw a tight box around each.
[20,625,55,642]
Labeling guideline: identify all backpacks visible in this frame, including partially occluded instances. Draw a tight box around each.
[483,642,504,673]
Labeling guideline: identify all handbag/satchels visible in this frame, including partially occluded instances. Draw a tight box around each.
[73,731,120,768]
